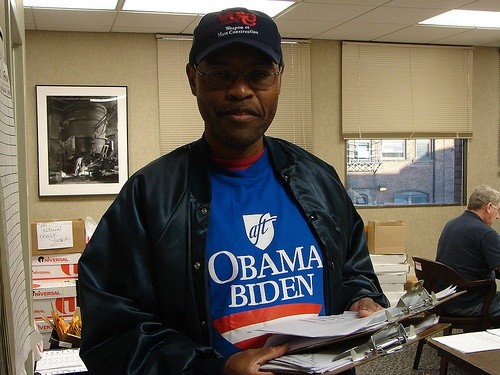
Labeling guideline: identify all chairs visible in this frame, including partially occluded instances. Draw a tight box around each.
[411,256,498,375]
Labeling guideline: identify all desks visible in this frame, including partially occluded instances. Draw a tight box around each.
[428,327,500,375]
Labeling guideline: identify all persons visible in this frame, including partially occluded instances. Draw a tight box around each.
[427,184,499,322]
[74,7,394,375]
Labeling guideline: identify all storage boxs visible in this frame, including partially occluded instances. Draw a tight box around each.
[368,219,407,255]
[369,254,408,264]
[377,272,408,284]
[372,264,411,274]
[28,218,87,349]
[382,290,407,301]
[380,284,405,292]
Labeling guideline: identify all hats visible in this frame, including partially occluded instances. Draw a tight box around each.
[189,7,282,66]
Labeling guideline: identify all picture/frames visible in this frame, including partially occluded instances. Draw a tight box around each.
[35,84,129,198]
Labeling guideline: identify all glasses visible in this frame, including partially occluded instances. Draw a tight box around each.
[194,65,282,90]
[485,201,500,215]
